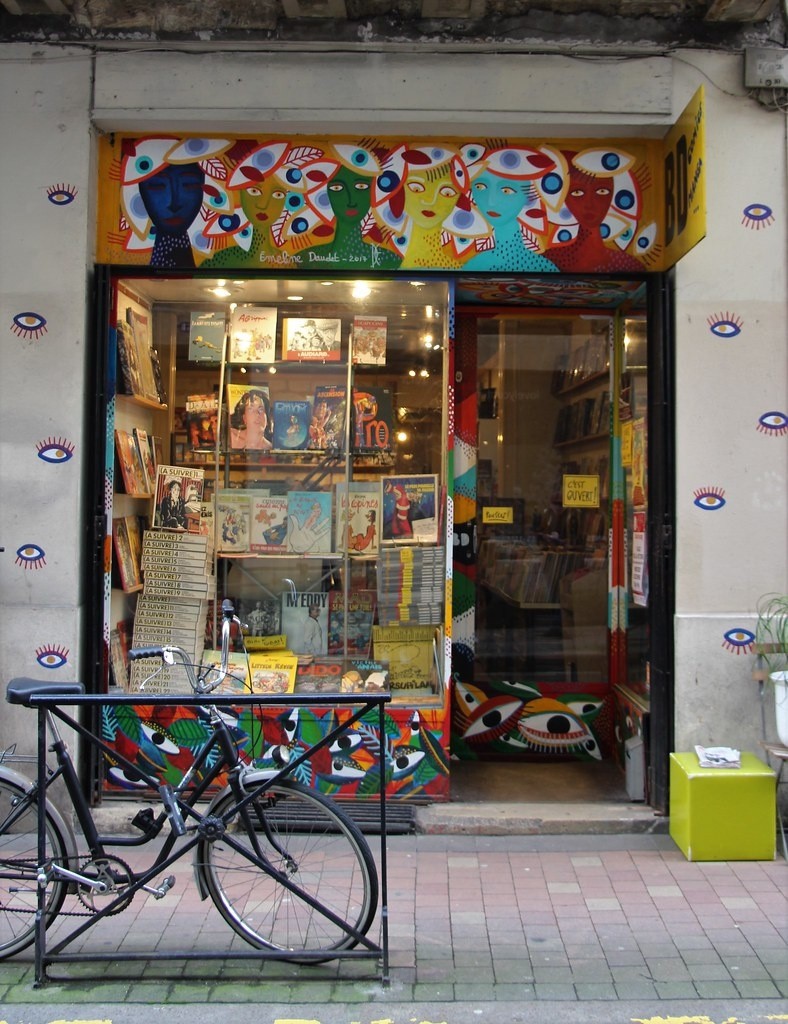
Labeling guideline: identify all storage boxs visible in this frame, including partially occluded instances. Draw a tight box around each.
[113,304,445,696]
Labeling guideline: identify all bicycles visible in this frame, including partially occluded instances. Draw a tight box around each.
[0,599,379,964]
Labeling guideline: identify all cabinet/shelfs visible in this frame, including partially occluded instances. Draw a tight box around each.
[468,311,646,683]
[109,287,449,803]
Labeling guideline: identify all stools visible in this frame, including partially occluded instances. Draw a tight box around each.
[669,750,778,862]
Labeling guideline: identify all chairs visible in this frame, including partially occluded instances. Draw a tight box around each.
[752,643,788,864]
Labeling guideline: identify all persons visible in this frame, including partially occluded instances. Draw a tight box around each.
[230,390,273,450]
[303,604,322,657]
[246,602,267,637]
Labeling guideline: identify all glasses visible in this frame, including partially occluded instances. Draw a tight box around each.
[313,609,320,612]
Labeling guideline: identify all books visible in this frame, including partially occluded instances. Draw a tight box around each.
[110,306,446,693]
[478,509,602,603]
[553,327,609,497]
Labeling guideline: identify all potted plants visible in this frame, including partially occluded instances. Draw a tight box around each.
[753,590,788,747]
